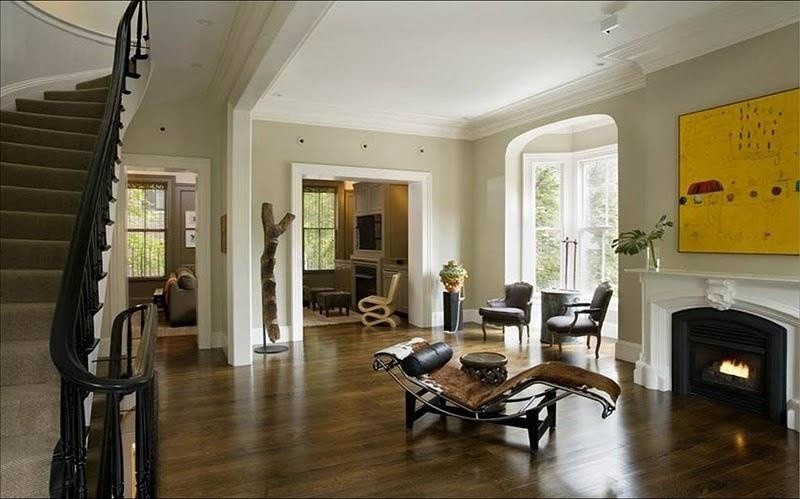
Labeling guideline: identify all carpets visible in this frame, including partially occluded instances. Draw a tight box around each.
[127,308,198,339]
[303,301,362,327]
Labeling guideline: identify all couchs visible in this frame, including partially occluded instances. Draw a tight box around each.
[166,267,198,327]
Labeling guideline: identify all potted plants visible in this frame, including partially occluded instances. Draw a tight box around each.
[611,213,673,271]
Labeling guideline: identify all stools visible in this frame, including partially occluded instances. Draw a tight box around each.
[152,287,166,311]
[316,291,352,317]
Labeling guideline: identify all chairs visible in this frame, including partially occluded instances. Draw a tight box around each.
[358,272,402,328]
[547,282,613,359]
[478,281,532,343]
[372,337,621,451]
